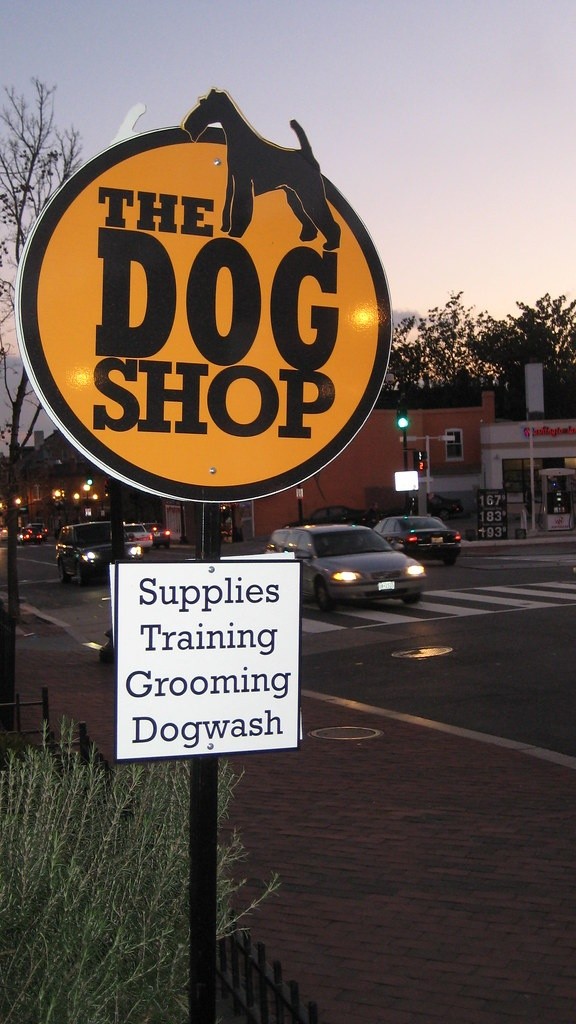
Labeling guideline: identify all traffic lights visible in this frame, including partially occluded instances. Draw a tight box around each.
[397,402,409,430]
[86,472,95,486]
[415,451,428,472]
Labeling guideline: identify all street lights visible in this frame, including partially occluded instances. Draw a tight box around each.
[400,434,456,496]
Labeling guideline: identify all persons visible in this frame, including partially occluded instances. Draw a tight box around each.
[363,501,378,528]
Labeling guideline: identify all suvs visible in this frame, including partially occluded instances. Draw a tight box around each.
[54,521,144,587]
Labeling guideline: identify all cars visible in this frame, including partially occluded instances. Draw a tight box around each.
[281,504,379,530]
[0,523,48,545]
[143,523,170,550]
[413,493,464,521]
[122,524,153,553]
[265,525,428,613]
[374,515,462,566]
